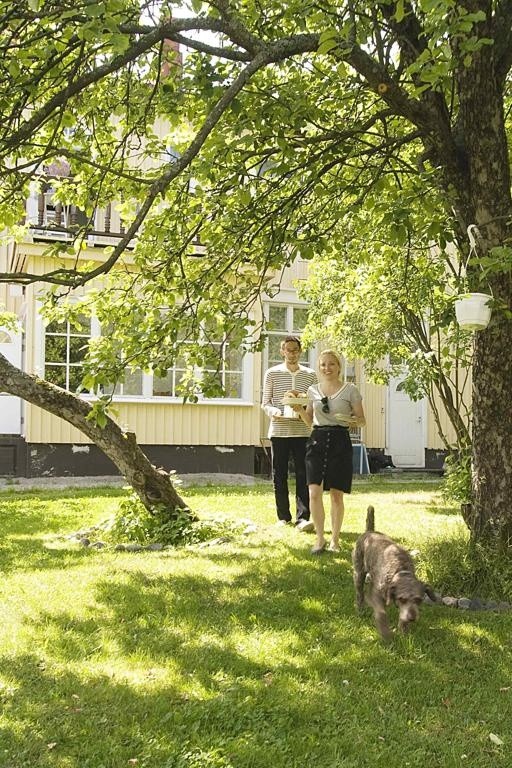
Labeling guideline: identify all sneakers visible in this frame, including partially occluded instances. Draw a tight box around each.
[276,520,314,532]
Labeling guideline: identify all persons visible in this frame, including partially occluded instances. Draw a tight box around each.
[259,334,319,532]
[289,348,368,556]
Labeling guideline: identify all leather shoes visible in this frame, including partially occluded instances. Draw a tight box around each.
[310,540,327,555]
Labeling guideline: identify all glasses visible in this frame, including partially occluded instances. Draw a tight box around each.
[321,397,330,414]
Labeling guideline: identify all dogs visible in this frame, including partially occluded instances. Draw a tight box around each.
[350,504,437,643]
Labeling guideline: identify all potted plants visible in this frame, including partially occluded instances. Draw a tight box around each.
[447,221,508,331]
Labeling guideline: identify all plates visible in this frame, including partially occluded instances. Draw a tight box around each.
[273,414,304,424]
[279,397,309,407]
[333,413,360,422]
